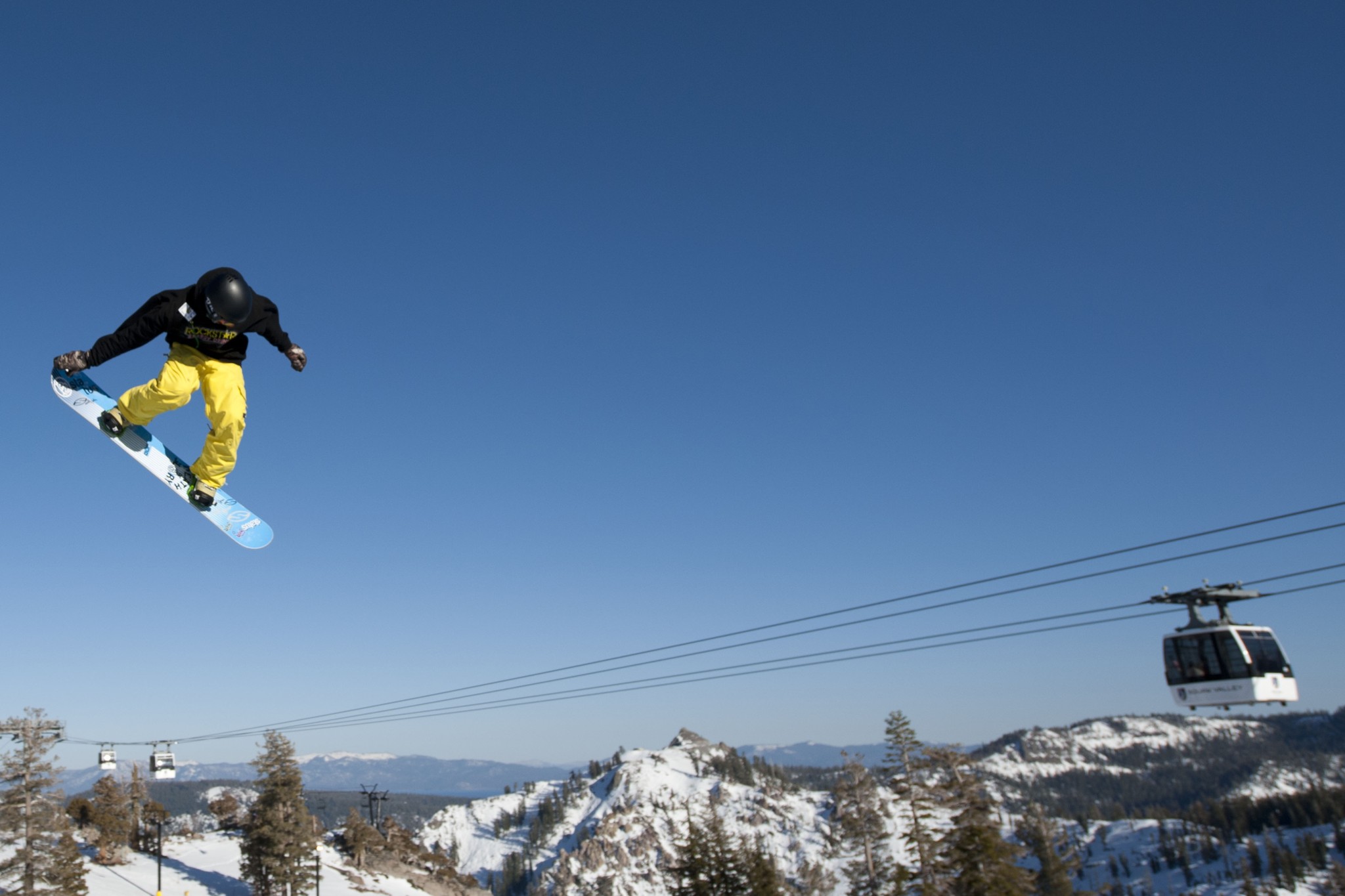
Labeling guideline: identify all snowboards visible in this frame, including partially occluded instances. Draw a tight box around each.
[50,366,274,549]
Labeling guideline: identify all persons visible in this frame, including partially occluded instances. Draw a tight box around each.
[53,268,307,506]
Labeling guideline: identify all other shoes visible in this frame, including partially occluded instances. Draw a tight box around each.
[103,405,133,436]
[189,476,216,506]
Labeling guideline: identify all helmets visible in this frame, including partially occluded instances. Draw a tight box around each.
[197,266,253,324]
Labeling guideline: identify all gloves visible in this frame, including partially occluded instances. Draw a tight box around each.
[284,343,307,372]
[52,349,90,375]
